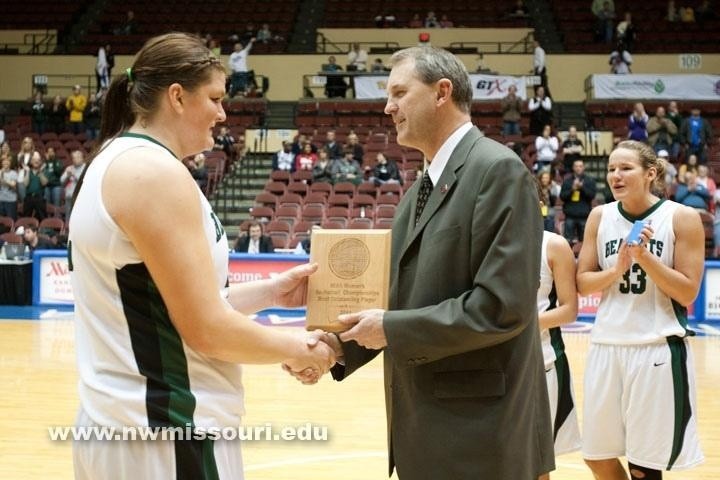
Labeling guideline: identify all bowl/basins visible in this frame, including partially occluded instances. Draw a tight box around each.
[3,244,25,260]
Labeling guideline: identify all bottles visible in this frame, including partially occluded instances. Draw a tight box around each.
[24,245,30,259]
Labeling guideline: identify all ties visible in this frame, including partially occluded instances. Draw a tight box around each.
[415,170,433,224]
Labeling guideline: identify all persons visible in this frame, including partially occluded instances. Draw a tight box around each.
[285,47,555,479]
[0,0,719,260]
[576,139,705,479]
[67,30,338,480]
[537,229,584,480]
